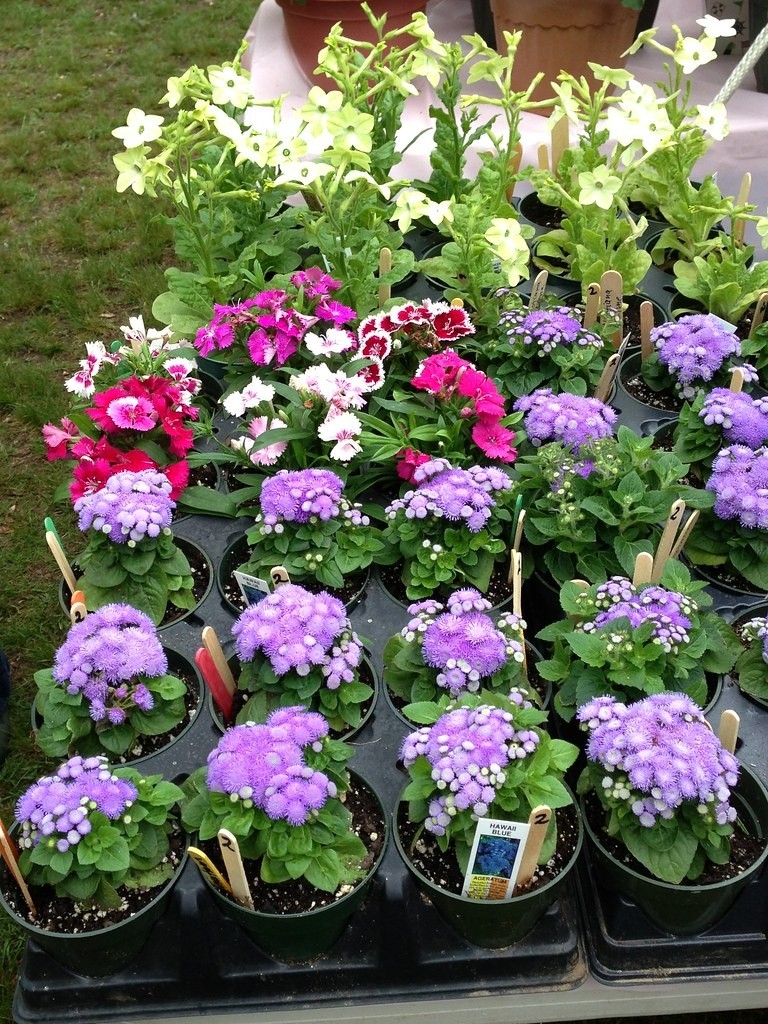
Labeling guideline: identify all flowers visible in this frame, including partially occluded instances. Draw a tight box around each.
[15,11,768,908]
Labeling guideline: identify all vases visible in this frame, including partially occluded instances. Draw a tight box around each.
[0,182,768,974]
[276,1,428,105]
[490,1,640,117]
